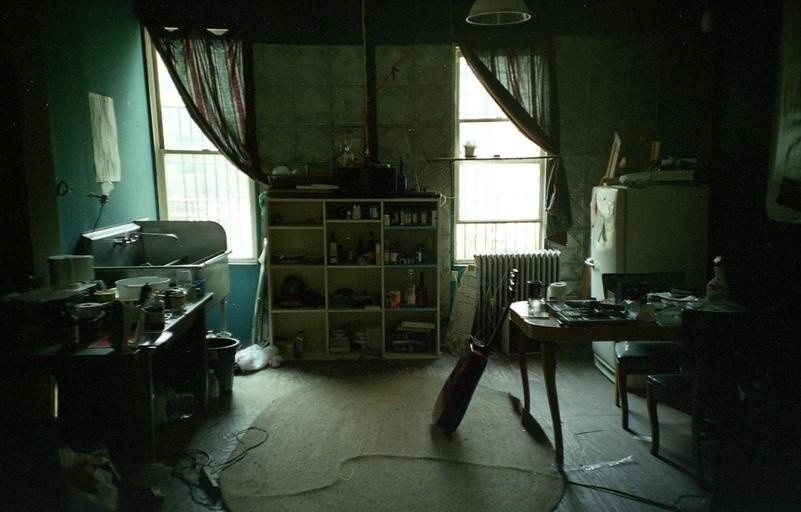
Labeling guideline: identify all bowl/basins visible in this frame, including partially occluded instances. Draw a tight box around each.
[115,276,171,300]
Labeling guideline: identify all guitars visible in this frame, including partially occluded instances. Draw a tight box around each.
[430,268,519,430]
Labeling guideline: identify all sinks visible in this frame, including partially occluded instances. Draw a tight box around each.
[92,254,184,268]
[166,249,232,267]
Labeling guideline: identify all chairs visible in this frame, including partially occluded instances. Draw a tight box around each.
[601,270,789,488]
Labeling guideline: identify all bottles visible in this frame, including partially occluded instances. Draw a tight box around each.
[403,269,427,307]
[396,156,407,197]
[328,205,437,265]
[286,332,306,360]
[203,368,220,412]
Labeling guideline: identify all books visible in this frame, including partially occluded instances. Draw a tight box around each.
[391,321,437,354]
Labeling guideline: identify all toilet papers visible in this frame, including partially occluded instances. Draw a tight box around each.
[546,281,567,306]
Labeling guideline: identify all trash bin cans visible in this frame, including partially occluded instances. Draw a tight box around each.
[206,338,240,418]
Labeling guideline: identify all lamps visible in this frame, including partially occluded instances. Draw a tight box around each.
[463,0,533,27]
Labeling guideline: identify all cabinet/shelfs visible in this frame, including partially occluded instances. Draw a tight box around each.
[264,188,444,361]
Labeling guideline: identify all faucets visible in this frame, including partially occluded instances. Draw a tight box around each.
[114,231,180,245]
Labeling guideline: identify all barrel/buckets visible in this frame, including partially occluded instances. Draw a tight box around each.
[205,333,243,410]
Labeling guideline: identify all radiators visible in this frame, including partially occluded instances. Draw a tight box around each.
[473,248,564,346]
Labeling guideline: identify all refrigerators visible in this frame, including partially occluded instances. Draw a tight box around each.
[584,185,710,391]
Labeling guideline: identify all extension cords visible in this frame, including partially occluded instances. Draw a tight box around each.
[201,466,220,495]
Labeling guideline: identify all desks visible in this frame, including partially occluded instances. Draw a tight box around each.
[25,290,218,464]
[506,289,801,464]
